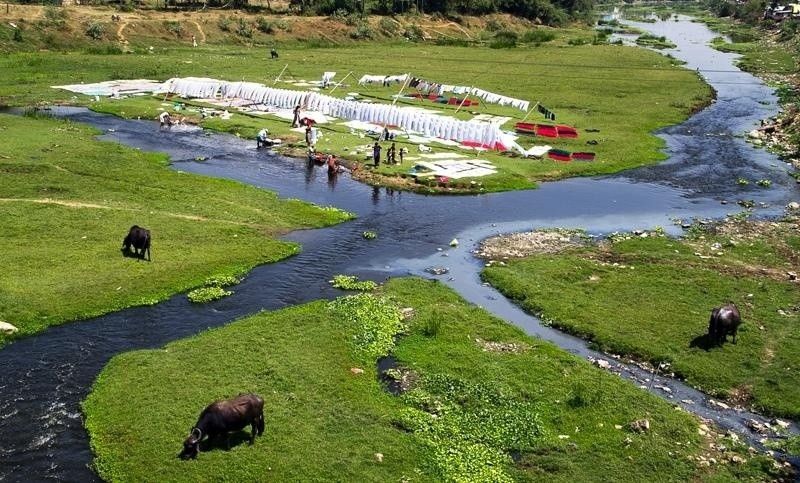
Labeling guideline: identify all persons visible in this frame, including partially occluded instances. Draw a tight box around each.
[160,106,403,175]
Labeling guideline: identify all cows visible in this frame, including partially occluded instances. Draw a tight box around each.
[182,392,266,462]
[122,224,154,261]
[270,49,280,59]
[707,301,743,349]
